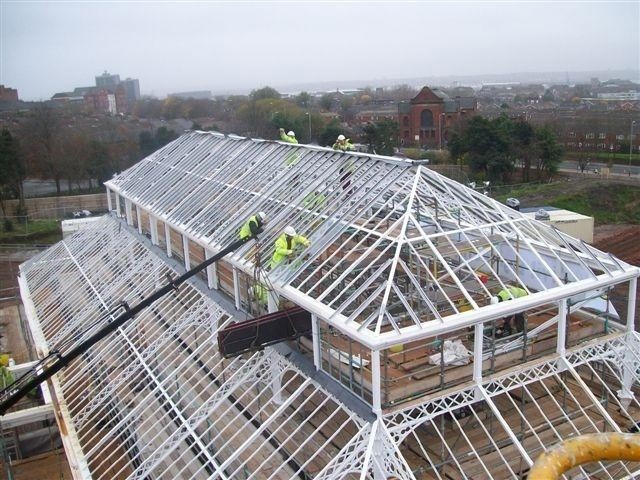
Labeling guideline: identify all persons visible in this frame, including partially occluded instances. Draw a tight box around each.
[279,127,299,189]
[271,227,311,269]
[332,134,356,199]
[248,286,267,311]
[302,186,326,226]
[490,288,528,334]
[238,212,266,241]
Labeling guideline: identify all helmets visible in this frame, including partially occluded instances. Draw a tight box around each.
[284,226,296,236]
[490,296,499,304]
[338,135,346,140]
[287,131,295,137]
[259,212,266,219]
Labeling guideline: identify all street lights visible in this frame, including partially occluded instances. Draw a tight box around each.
[470,181,490,196]
[439,112,445,154]
[390,100,406,154]
[628,121,636,178]
[306,112,313,145]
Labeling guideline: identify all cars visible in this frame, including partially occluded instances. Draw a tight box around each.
[54,208,109,230]
